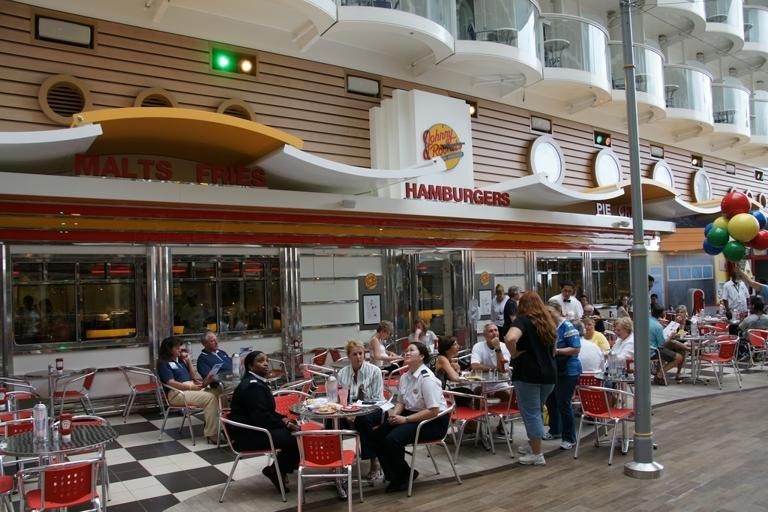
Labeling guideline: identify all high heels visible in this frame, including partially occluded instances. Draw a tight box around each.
[207,434,227,445]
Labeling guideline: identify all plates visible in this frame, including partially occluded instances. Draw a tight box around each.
[340,406,362,412]
[606,306,616,309]
[312,408,337,415]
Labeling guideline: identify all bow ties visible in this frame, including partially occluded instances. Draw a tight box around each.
[563,299,571,302]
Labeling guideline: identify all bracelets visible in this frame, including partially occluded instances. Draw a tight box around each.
[405,416,409,422]
[286,420,291,426]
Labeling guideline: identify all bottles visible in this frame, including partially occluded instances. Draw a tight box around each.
[433,338,438,351]
[338,383,342,388]
[52,425,59,440]
[60,413,73,440]
[55,358,64,373]
[326,376,336,403]
[7,393,16,412]
[625,358,635,377]
[609,311,612,318]
[0,387,7,410]
[231,354,240,376]
[607,350,617,378]
[698,326,704,336]
[488,368,499,379]
[30,403,47,443]
[48,363,54,374]
[617,388,622,410]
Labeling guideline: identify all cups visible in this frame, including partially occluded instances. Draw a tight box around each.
[338,389,349,406]
[474,369,482,378]
[678,329,684,339]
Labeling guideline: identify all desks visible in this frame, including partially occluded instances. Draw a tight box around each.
[544,39,571,67]
[714,107,739,123]
[489,26,518,45]
[664,84,679,108]
[633,72,651,91]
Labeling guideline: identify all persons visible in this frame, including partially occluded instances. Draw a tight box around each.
[155,335,228,445]
[17,294,40,337]
[196,329,234,388]
[369,263,768,424]
[225,350,304,496]
[325,338,386,482]
[502,290,559,469]
[35,296,68,340]
[544,297,584,452]
[372,340,449,495]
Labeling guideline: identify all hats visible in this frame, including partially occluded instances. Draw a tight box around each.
[509,286,524,295]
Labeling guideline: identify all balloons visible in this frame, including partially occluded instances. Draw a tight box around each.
[700,189,768,264]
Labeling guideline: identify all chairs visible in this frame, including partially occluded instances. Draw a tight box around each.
[364,335,638,497]
[217,410,288,503]
[467,22,495,40]
[0,356,120,512]
[585,309,767,389]
[291,429,364,512]
[234,335,364,428]
[118,335,234,449]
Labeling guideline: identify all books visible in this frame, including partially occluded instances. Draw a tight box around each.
[350,398,396,412]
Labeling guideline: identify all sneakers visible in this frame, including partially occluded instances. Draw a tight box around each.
[372,464,381,479]
[654,375,665,384]
[386,470,418,492]
[518,444,546,465]
[560,441,576,450]
[543,432,554,439]
[262,466,290,493]
